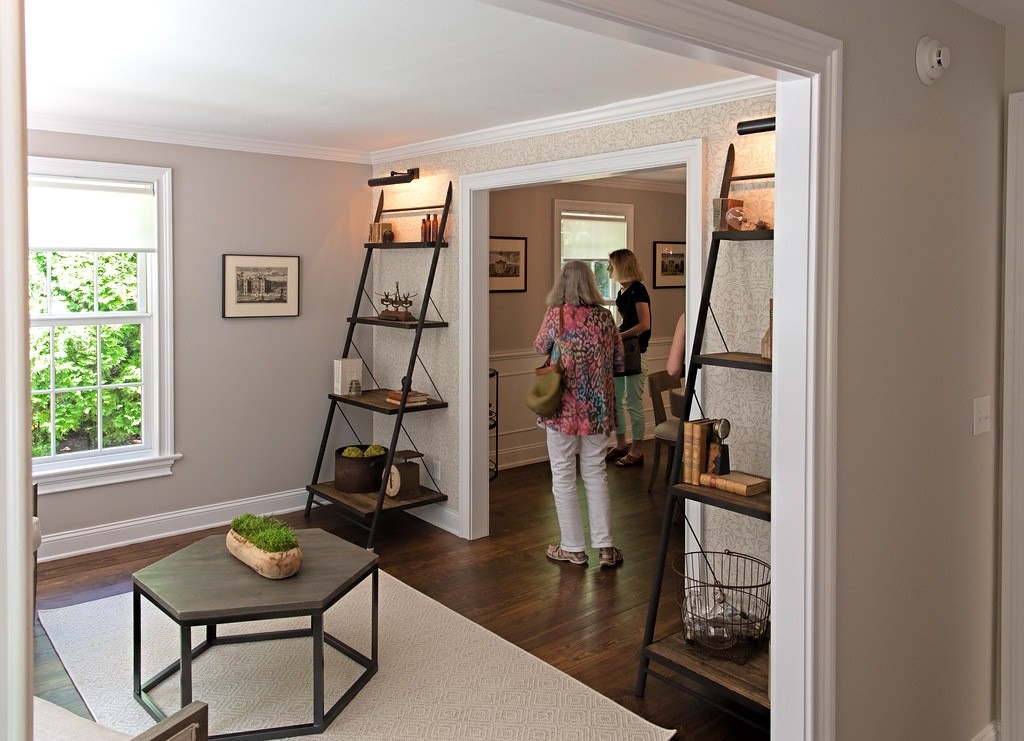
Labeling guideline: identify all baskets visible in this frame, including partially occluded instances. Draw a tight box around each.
[671,548,770,659]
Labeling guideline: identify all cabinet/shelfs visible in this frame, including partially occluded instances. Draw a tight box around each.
[304,181,452,554]
[636,143,774,732]
[490,369,499,481]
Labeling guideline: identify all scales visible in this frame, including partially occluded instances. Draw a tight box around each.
[381,450,424,501]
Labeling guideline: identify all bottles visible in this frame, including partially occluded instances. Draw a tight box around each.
[348,380,362,399]
[419,213,439,241]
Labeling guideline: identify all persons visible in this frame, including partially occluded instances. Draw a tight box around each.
[534,261,626,566]
[661,310,686,389]
[602,249,651,467]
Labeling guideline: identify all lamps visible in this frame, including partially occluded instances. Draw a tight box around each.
[368,167,420,188]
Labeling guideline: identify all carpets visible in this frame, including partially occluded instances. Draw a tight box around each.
[37,569,676,741]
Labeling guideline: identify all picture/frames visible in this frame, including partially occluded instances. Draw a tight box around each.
[489,235,527,293]
[222,254,300,317]
[652,240,686,288]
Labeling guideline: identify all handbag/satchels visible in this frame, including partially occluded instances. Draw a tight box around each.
[614,338,641,377]
[526,363,562,416]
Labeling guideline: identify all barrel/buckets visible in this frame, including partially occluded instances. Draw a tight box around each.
[335,444,389,493]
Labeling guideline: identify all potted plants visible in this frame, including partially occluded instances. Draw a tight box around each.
[226,515,303,580]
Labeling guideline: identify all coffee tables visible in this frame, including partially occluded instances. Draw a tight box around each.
[131,530,379,741]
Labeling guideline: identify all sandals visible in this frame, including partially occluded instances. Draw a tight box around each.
[606,447,629,460]
[546,545,589,564]
[616,454,644,466]
[600,547,624,565]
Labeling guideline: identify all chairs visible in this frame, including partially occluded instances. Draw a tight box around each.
[33,698,206,741]
[647,368,681,490]
